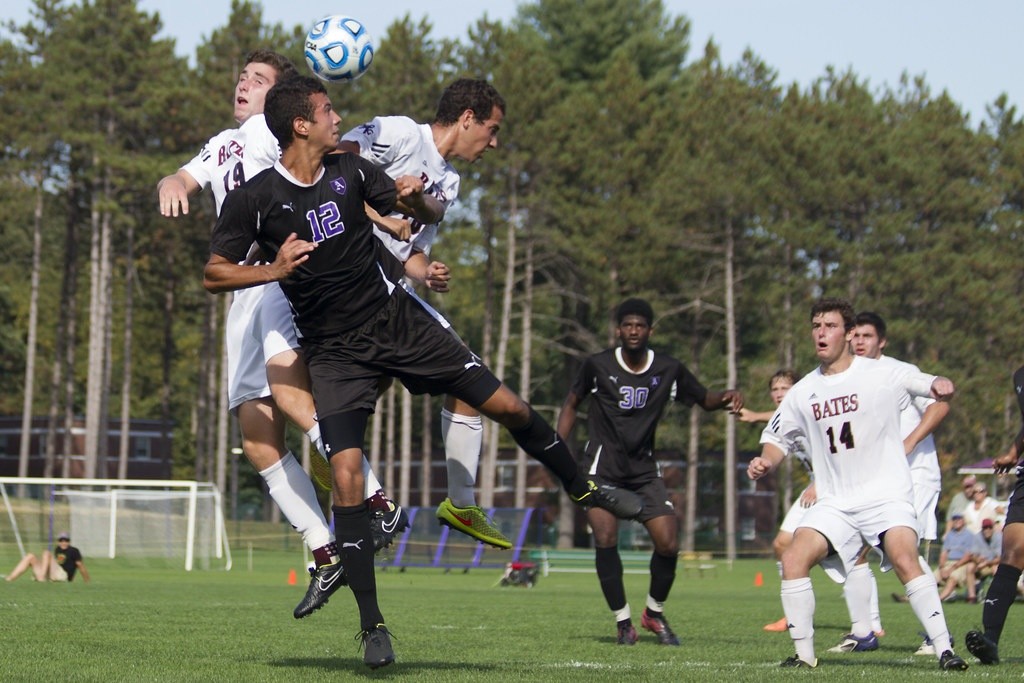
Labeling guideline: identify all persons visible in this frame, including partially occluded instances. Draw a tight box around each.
[1,532,91,584]
[887,476,1024,604]
[727,295,971,669]
[155,46,654,667]
[553,292,742,647]
[965,362,1024,664]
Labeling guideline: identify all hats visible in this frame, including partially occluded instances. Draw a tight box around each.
[963,476,975,486]
[952,512,963,519]
[57,532,69,540]
[982,520,992,527]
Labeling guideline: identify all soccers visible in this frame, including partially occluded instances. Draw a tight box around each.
[305,16,376,82]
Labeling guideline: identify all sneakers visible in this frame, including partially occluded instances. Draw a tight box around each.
[570,477,645,518]
[914,631,954,655]
[642,608,678,646]
[939,650,969,670]
[309,441,332,492]
[966,630,999,665]
[437,497,512,550]
[764,617,788,632]
[373,499,409,550]
[780,654,818,669]
[828,631,878,654]
[294,558,350,619]
[355,623,396,669]
[616,616,638,645]
